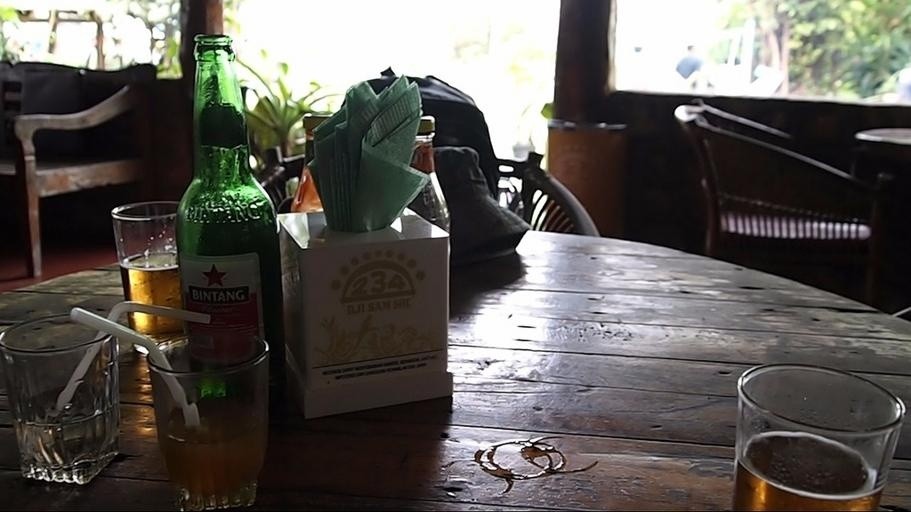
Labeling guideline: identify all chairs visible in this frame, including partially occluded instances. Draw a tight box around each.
[260,155,600,236]
[0,61,154,276]
[674,105,884,300]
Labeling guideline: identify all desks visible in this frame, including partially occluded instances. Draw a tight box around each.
[853,126,911,149]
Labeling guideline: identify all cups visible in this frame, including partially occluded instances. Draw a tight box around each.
[730,363,907,512]
[147,334,272,512]
[0,313,120,484]
[110,202,184,338]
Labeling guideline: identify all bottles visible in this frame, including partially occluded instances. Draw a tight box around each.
[172,33,286,397]
[285,115,451,238]
